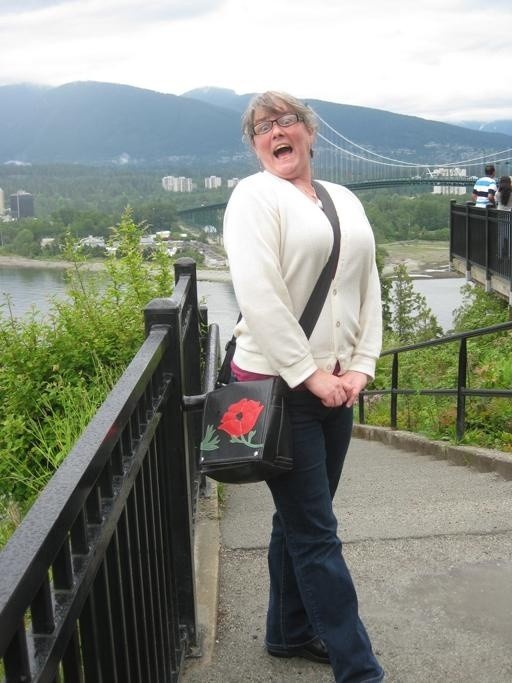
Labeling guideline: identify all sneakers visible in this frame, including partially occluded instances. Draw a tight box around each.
[267,636,328,663]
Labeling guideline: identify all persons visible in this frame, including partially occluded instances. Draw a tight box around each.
[471,164,497,264]
[493,175,512,263]
[221,87,387,682]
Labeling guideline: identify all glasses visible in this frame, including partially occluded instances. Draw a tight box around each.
[251,112,305,138]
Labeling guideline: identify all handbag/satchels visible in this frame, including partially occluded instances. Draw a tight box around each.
[198,378,291,486]
